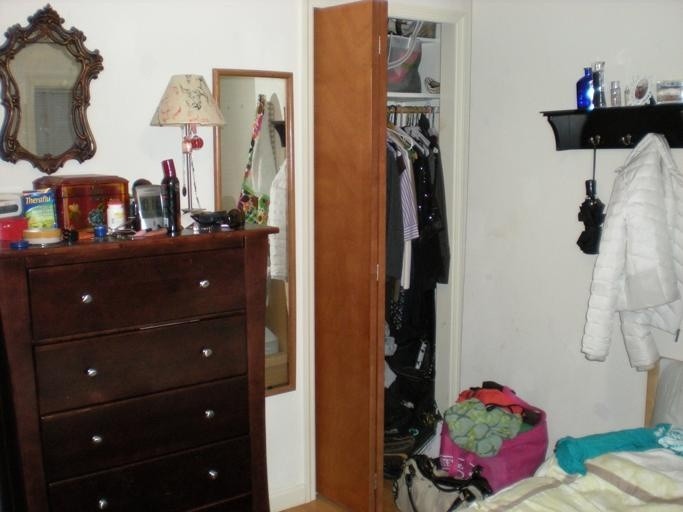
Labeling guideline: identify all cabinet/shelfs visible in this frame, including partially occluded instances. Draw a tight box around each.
[315,0,465,512]
[2,224,279,511]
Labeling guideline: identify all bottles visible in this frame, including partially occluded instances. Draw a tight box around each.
[161,158,185,233]
[107,200,125,234]
[576,60,622,109]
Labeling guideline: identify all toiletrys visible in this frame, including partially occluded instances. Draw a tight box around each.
[610,81,621,107]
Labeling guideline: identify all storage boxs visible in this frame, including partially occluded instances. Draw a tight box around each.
[31,174,131,237]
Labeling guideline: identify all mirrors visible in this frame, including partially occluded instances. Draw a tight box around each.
[0,4,104,174]
[213,70,298,398]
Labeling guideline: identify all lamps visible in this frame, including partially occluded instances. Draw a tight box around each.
[150,75,228,228]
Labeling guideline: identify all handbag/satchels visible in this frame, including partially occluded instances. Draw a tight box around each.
[386,33,423,94]
[392,453,495,511]
[388,17,437,39]
[437,382,552,496]
[236,184,271,227]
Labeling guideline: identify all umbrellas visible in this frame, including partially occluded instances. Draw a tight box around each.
[576,179,606,255]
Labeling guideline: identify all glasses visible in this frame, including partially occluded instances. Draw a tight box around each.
[225,205,246,229]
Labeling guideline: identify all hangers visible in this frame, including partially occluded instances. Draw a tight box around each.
[385,105,438,160]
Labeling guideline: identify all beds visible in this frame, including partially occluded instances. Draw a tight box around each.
[469,356,683,511]
